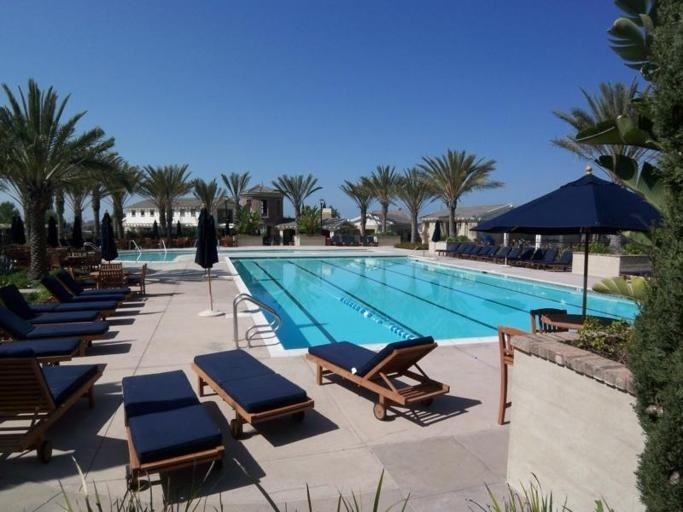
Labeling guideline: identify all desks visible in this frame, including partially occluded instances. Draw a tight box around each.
[541,314,629,330]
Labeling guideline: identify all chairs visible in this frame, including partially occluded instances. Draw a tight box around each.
[497,308,568,425]
[0,264,147,463]
[305,335,451,420]
[329,235,378,246]
[125,370,233,491]
[192,347,316,436]
[435,242,572,272]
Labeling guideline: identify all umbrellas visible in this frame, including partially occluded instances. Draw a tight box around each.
[469,166,665,316]
[195,202,219,310]
[100,212,118,264]
